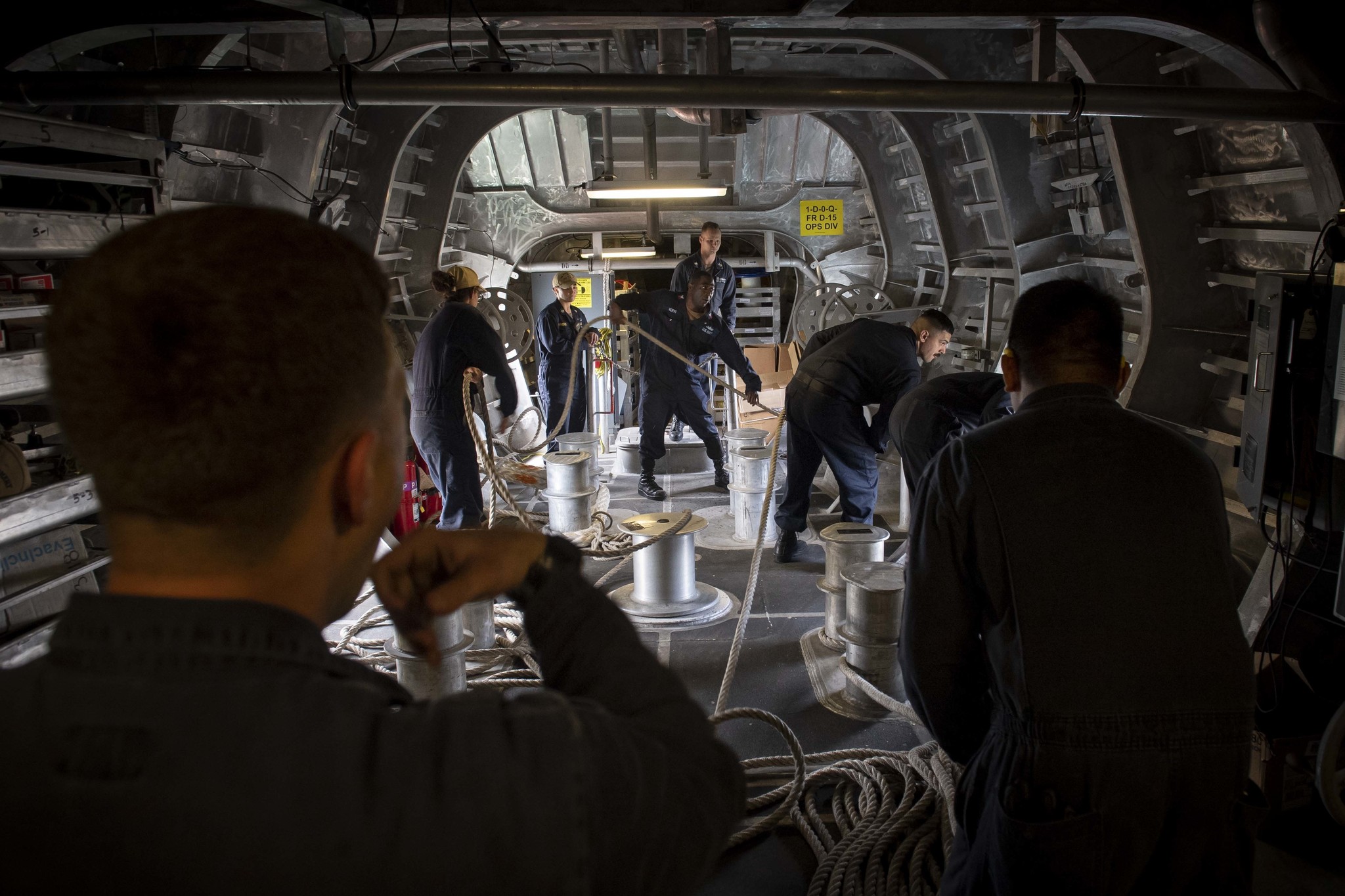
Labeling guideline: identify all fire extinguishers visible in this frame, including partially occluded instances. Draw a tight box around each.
[414,442,441,522]
[390,460,420,542]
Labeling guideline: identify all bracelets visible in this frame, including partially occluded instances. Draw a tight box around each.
[508,536,580,612]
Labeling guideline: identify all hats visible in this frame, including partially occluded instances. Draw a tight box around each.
[552,271,581,289]
[443,265,488,295]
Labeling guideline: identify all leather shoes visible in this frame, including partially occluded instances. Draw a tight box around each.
[669,415,686,441]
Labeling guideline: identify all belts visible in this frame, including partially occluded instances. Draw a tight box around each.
[795,370,850,402]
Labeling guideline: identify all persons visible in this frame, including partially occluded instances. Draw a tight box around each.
[899,277,1259,896]
[535,270,602,465]
[773,309,954,563]
[889,371,1016,497]
[608,270,762,501]
[669,222,737,441]
[0,204,748,896]
[409,264,518,530]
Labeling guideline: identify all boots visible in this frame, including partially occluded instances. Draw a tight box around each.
[638,466,667,501]
[773,529,807,563]
[712,457,729,489]
